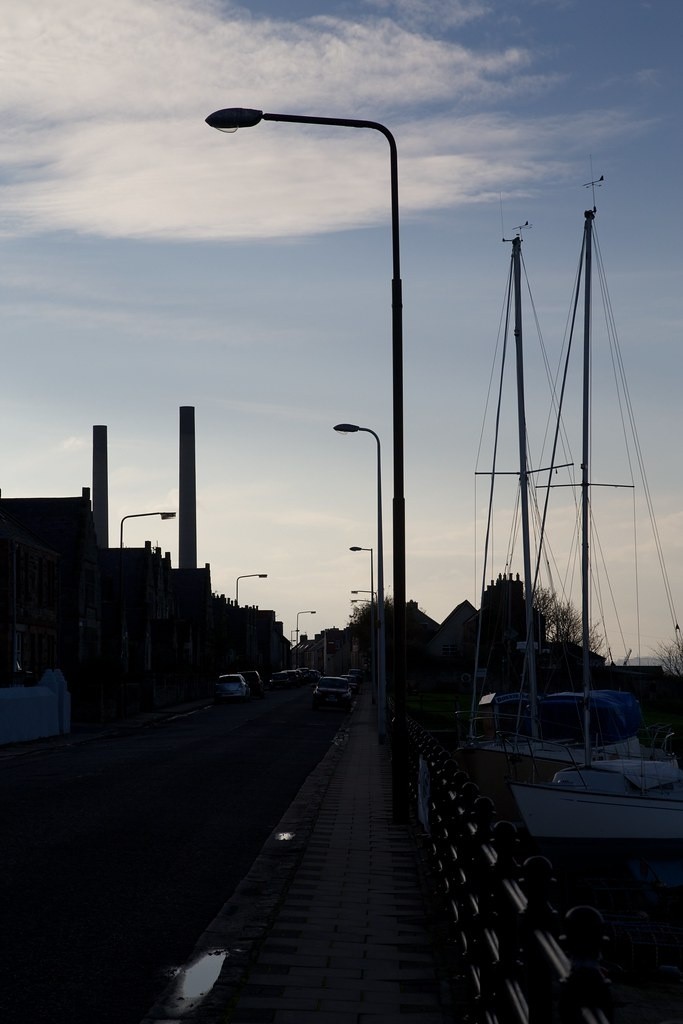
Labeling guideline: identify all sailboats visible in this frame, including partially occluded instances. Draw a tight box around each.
[454,175,683,843]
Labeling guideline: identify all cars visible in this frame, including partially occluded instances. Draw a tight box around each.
[214,674,251,702]
[340,675,360,694]
[271,668,321,690]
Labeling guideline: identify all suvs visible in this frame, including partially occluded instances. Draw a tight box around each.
[237,671,265,698]
[311,675,353,714]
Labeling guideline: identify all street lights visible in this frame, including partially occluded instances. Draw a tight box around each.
[237,574,269,605]
[348,547,377,706]
[333,424,388,749]
[121,512,176,721]
[206,104,415,828]
[296,610,316,668]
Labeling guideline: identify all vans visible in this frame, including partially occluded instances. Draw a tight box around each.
[348,668,361,676]
